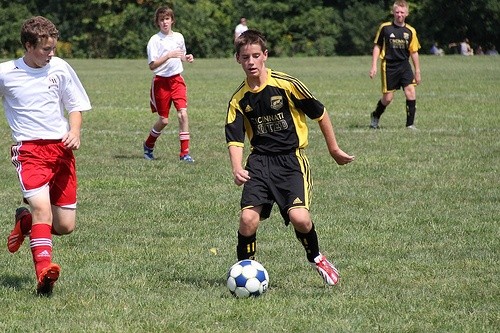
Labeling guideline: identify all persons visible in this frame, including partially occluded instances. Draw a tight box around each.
[0,16,92,296]
[142,6,194,162]
[225,29,355,285]
[431,41,444,56]
[460,38,498,56]
[369,0,421,129]
[232,18,248,58]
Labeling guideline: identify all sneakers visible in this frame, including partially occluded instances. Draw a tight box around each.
[37,263,60,295]
[180,154,194,162]
[143,144,155,161]
[305,251,340,286]
[7,207,29,253]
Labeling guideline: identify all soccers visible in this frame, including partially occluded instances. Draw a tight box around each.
[225,258,271,300]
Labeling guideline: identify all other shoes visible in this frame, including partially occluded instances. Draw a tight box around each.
[408,125,417,129]
[370,111,378,128]
